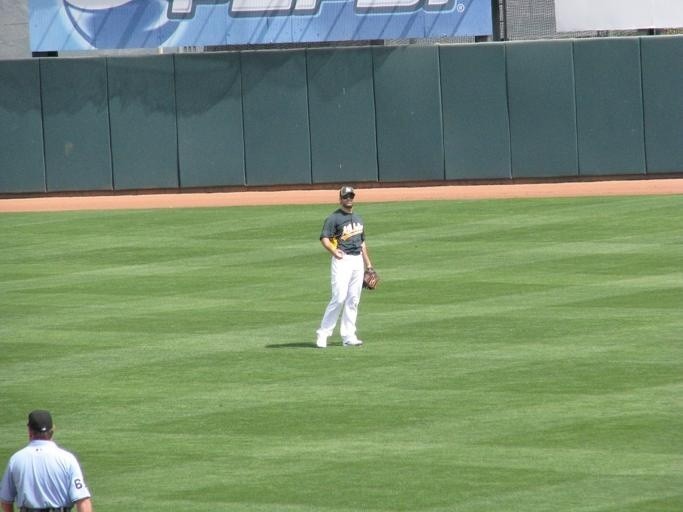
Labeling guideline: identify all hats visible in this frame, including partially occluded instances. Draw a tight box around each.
[340,187,355,198]
[27,410,52,431]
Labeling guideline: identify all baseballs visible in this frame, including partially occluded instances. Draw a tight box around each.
[337,251,342,256]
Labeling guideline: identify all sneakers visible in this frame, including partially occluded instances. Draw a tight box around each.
[316,334,327,348]
[343,337,363,346]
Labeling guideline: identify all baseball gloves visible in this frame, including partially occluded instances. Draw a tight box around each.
[363,268,378,288]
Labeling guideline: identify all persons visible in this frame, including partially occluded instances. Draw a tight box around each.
[315,184,379,349]
[1,409,92,512]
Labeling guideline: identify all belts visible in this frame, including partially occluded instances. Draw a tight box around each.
[20,507,71,512]
[347,251,360,255]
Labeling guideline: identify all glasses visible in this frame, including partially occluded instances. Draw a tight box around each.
[344,194,354,199]
